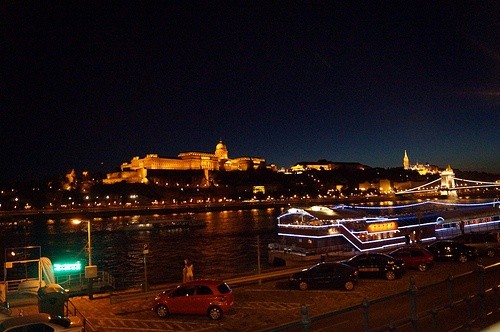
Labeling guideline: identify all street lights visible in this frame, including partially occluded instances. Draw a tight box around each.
[72,219,93,300]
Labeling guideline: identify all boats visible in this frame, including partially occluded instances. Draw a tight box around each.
[0,245,116,299]
[267,197,500,267]
[137,214,209,230]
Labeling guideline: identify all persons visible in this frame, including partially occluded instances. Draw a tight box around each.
[460,220,466,234]
[404,229,421,245]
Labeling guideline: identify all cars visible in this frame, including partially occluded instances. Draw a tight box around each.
[287,262,360,291]
[488,231,500,244]
[150,279,234,320]
[336,250,408,281]
[387,246,435,272]
[424,239,479,264]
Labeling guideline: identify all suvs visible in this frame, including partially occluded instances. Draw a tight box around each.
[451,231,499,258]
